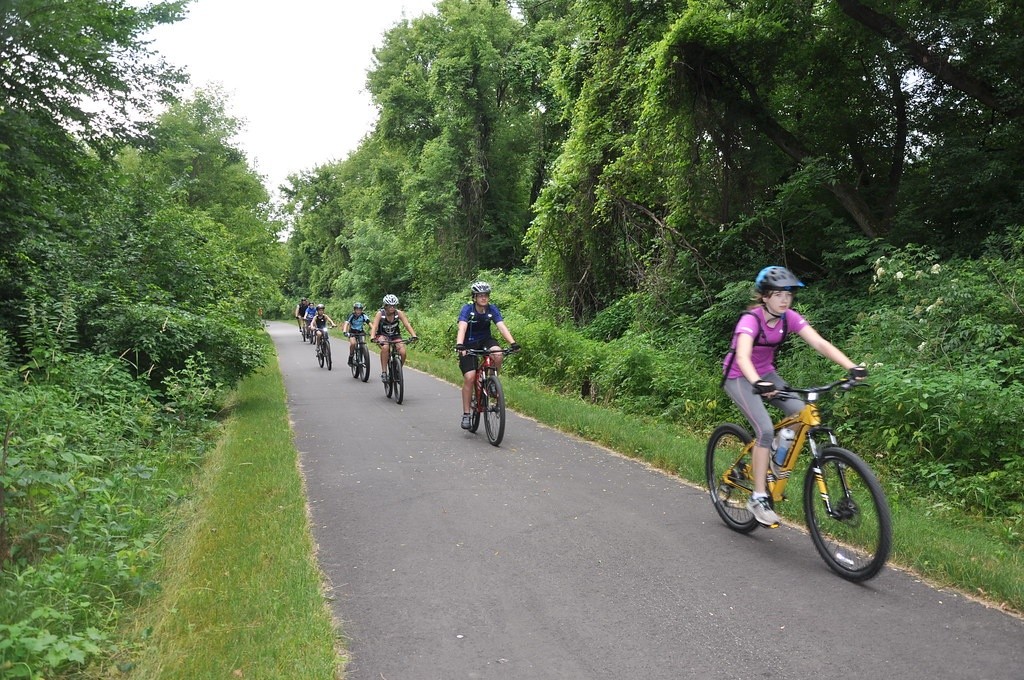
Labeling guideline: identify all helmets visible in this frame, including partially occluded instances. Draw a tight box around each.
[383,294,399,306]
[754,265,805,296]
[353,302,364,310]
[301,297,306,301]
[470,281,491,294]
[315,304,325,311]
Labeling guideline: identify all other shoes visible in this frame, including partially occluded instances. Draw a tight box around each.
[315,349,319,357]
[381,372,389,383]
[348,356,353,367]
[300,328,302,332]
[307,333,311,338]
[396,370,401,384]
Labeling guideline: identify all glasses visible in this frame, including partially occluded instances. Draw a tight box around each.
[387,305,396,309]
[475,293,489,298]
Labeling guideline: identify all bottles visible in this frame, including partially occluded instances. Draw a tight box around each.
[772,428,795,466]
[769,433,780,459]
[478,370,483,391]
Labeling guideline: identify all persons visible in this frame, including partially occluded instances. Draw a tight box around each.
[454,282,521,429]
[723,266,868,526]
[343,302,374,367]
[370,294,419,383]
[296,297,337,351]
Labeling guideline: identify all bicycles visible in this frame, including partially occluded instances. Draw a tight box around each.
[296,315,307,342]
[459,347,518,446]
[346,332,371,382]
[375,337,416,405]
[314,326,336,371]
[707,376,891,582]
[303,317,317,345]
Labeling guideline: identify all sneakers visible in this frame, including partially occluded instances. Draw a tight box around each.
[769,435,783,479]
[745,496,780,526]
[460,415,471,429]
[489,383,497,398]
